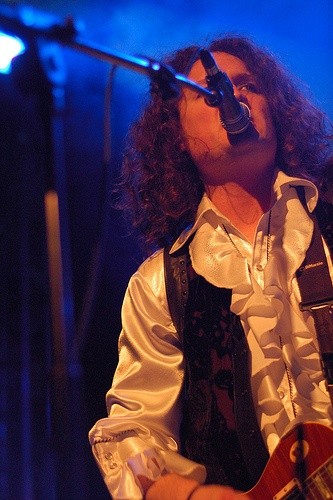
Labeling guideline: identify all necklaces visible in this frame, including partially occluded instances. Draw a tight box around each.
[223,210,271,272]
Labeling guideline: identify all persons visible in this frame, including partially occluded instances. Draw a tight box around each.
[87,35,333,500]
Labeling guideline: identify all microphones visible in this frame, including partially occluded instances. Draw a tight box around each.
[199,50,259,148]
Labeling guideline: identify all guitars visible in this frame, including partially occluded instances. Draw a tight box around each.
[239,423,333,500]
